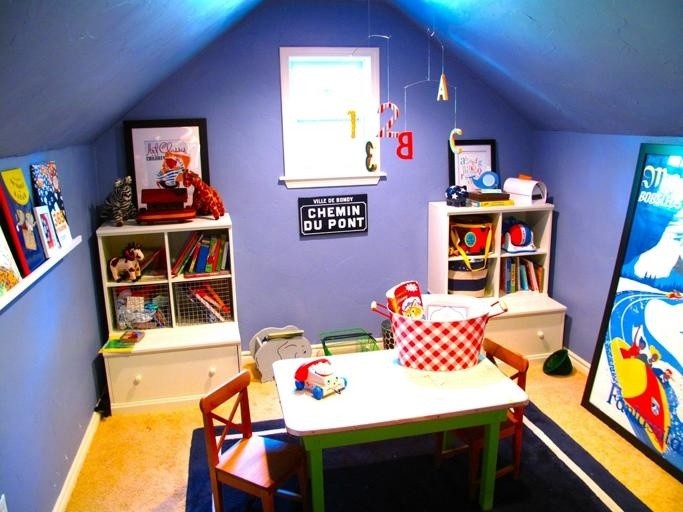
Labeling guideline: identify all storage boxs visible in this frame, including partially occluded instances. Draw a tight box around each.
[449,261,489,297]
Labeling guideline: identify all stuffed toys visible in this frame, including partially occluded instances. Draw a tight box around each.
[100,175,138,226]
[182,168,224,220]
[108,242,144,282]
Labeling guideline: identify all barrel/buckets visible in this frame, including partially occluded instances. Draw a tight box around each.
[371,297,505,373]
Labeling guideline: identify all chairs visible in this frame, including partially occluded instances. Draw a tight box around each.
[199,370,308,512]
[435,338,529,500]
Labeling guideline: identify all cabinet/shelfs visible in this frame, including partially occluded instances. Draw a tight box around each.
[96,211,241,333]
[481,291,567,360]
[100,323,244,416]
[428,202,555,296]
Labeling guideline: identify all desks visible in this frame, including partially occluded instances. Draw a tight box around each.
[272,348,529,511]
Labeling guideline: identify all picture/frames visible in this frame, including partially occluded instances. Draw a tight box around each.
[122,118,210,218]
[581,142,683,484]
[447,139,495,187]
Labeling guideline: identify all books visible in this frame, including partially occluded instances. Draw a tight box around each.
[0,168,50,276]
[0,224,22,299]
[385,280,425,319]
[98,335,136,353]
[120,331,144,343]
[30,161,72,249]
[171,232,230,278]
[502,257,543,293]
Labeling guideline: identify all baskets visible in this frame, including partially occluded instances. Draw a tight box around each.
[108,282,173,331]
[172,276,234,327]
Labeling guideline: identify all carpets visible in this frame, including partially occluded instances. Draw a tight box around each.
[186,400,655,512]
